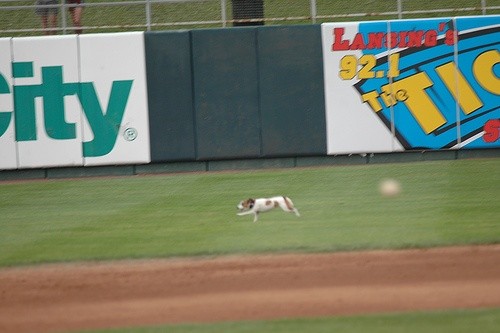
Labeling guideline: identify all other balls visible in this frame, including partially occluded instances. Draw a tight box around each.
[380,179,399,197]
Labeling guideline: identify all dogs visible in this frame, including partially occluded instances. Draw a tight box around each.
[236,195,301,223]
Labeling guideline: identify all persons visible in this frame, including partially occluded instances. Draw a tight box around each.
[35,0,57,35]
[66,0,83,34]
